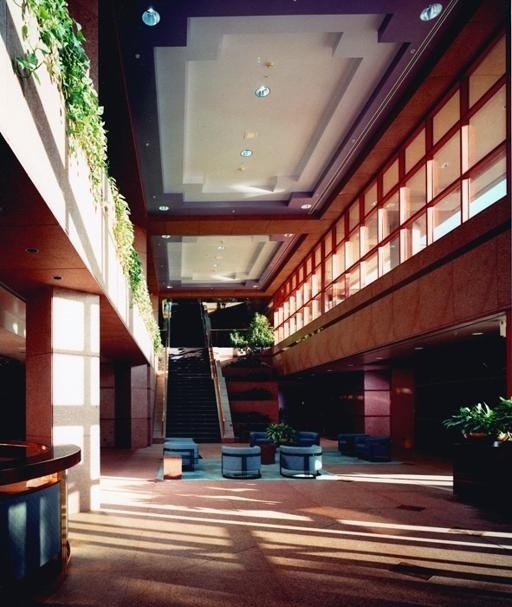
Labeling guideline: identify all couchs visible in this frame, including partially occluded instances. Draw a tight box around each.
[250,430,320,447]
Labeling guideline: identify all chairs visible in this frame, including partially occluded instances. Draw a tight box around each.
[221,445,262,479]
[279,444,323,479]
[163,438,199,471]
[338,432,392,463]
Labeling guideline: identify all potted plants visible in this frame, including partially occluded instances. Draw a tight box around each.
[441,393,512,513]
[263,421,298,444]
